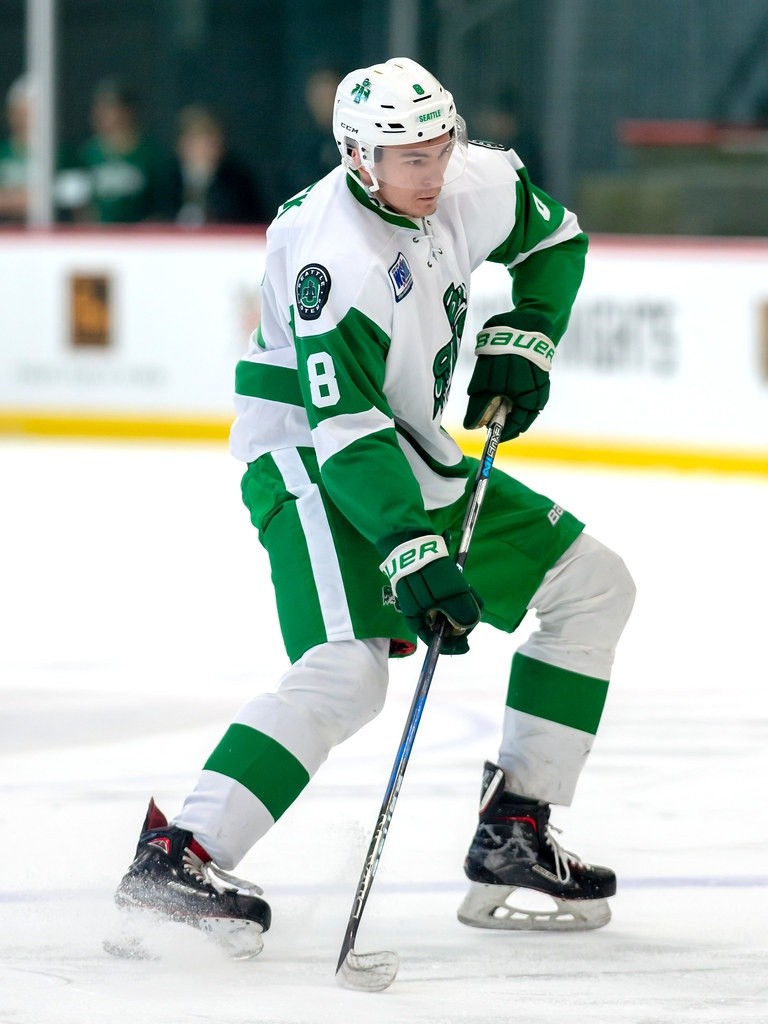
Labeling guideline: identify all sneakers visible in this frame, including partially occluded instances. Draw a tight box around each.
[455,759,617,931]
[104,798,273,958]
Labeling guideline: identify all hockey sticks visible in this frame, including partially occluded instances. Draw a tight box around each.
[330,398,509,994]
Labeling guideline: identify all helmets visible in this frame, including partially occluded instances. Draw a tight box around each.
[332,56,458,172]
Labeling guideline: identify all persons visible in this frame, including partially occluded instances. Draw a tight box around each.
[0,67,340,228]
[114,57,637,964]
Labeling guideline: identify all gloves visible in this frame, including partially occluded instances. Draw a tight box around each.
[462,312,557,441]
[376,530,485,656]
